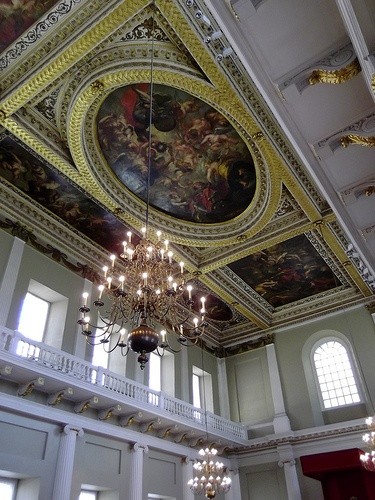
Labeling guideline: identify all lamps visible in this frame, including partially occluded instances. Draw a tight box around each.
[76,7,208,369]
[315,220,375,472]
[187,336,233,500]
